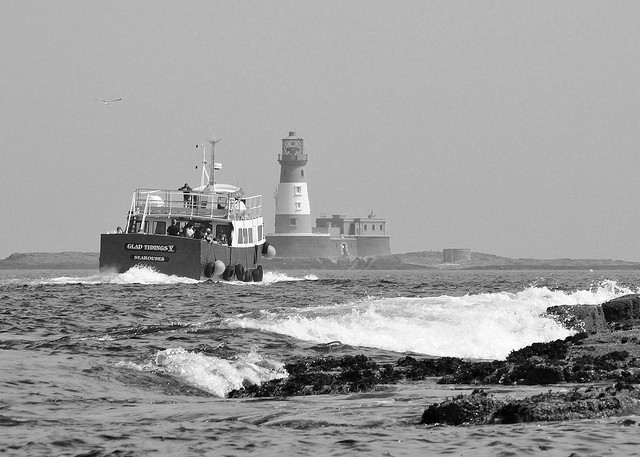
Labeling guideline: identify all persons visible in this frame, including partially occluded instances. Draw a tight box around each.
[217,228,225,241]
[197,225,203,237]
[167,219,179,237]
[204,227,211,239]
[184,223,196,237]
[178,183,192,208]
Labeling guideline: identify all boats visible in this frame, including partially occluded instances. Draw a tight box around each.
[99,132,276,283]
